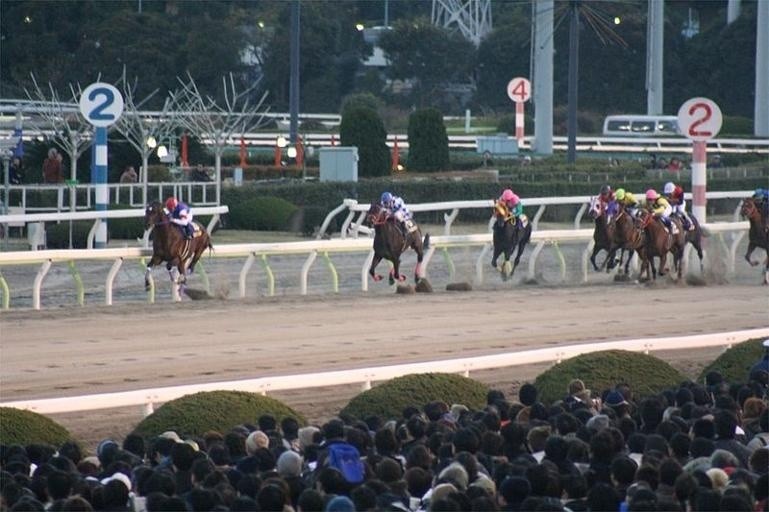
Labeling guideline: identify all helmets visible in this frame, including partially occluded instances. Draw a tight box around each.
[382,192,393,203]
[600,185,611,193]
[503,189,515,200]
[646,189,661,200]
[616,188,625,200]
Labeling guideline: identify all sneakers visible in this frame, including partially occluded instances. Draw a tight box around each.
[689,225,696,231]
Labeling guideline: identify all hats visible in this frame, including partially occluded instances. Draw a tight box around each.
[605,391,628,408]
[469,475,495,499]
[100,471,132,491]
[298,427,326,449]
[625,480,651,496]
[607,456,639,476]
[664,182,676,194]
[705,467,730,489]
[710,448,739,468]
[744,399,765,417]
[762,340,769,347]
[586,414,610,436]
[431,483,458,506]
[247,430,270,452]
[159,431,179,442]
[439,463,469,489]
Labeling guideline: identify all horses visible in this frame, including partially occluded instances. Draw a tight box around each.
[669,205,710,279]
[491,197,532,282]
[143,201,217,298]
[632,204,685,281]
[365,200,430,285]
[599,200,649,281]
[588,193,634,274]
[739,196,769,269]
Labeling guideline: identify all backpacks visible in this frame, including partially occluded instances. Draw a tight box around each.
[326,443,366,484]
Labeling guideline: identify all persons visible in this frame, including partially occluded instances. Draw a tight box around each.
[1,430,249,512]
[752,188,769,220]
[380,191,413,236]
[648,153,693,170]
[121,165,138,183]
[499,189,524,228]
[479,150,494,166]
[486,371,769,512]
[749,340,769,381]
[190,162,213,183]
[41,148,70,184]
[243,401,504,512]
[598,181,697,235]
[163,196,196,241]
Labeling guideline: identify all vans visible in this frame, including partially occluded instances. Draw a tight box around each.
[602,114,684,139]
